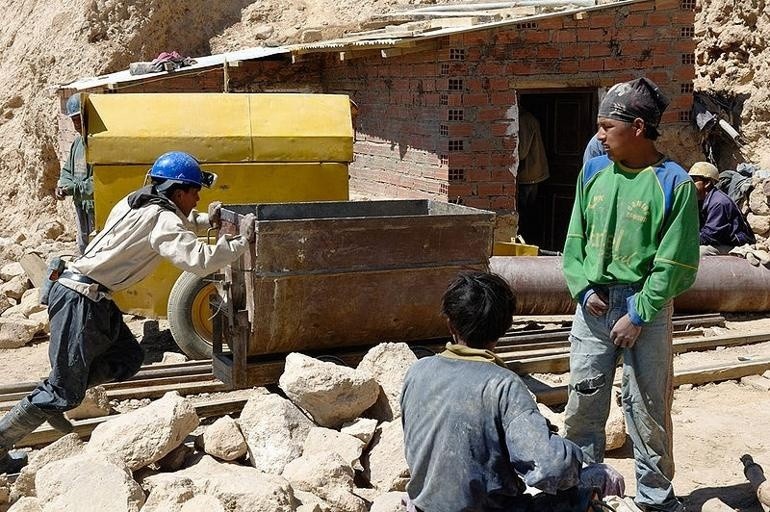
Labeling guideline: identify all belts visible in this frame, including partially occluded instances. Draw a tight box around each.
[60,271,114,295]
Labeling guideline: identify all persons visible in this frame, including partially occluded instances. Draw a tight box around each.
[583,133,609,167]
[518,102,550,245]
[561,77,699,512]
[0,150,258,474]
[396,269,602,512]
[688,162,756,257]
[54,93,95,253]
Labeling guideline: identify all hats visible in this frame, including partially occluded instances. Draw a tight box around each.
[598,76,671,128]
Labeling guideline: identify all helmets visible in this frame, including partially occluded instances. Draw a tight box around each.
[66,92,81,117]
[146,150,219,189]
[687,161,720,183]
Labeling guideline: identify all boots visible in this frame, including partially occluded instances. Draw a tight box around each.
[0,395,52,474]
[48,412,72,434]
